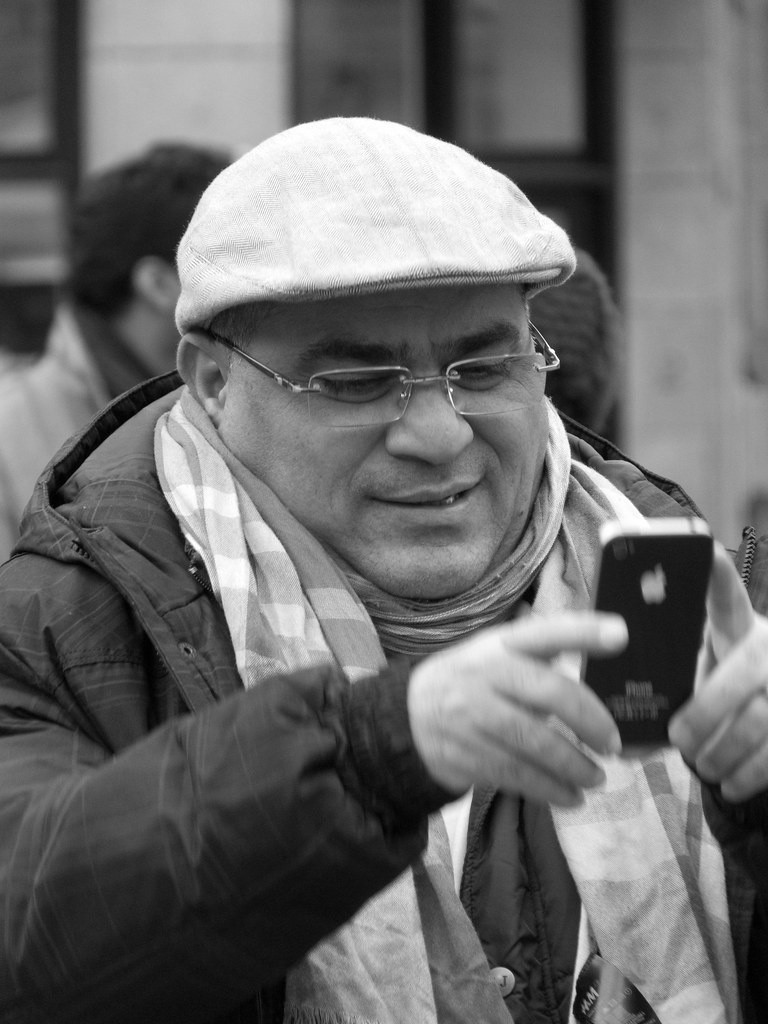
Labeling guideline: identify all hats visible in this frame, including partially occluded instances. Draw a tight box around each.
[172,112,576,340]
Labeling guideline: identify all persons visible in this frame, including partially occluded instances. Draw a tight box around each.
[0,116,768,1024]
[0,142,233,563]
[528,250,622,432]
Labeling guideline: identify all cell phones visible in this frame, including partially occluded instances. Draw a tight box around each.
[570,517,713,752]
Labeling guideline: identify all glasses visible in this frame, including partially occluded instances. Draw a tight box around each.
[198,319,563,429]
